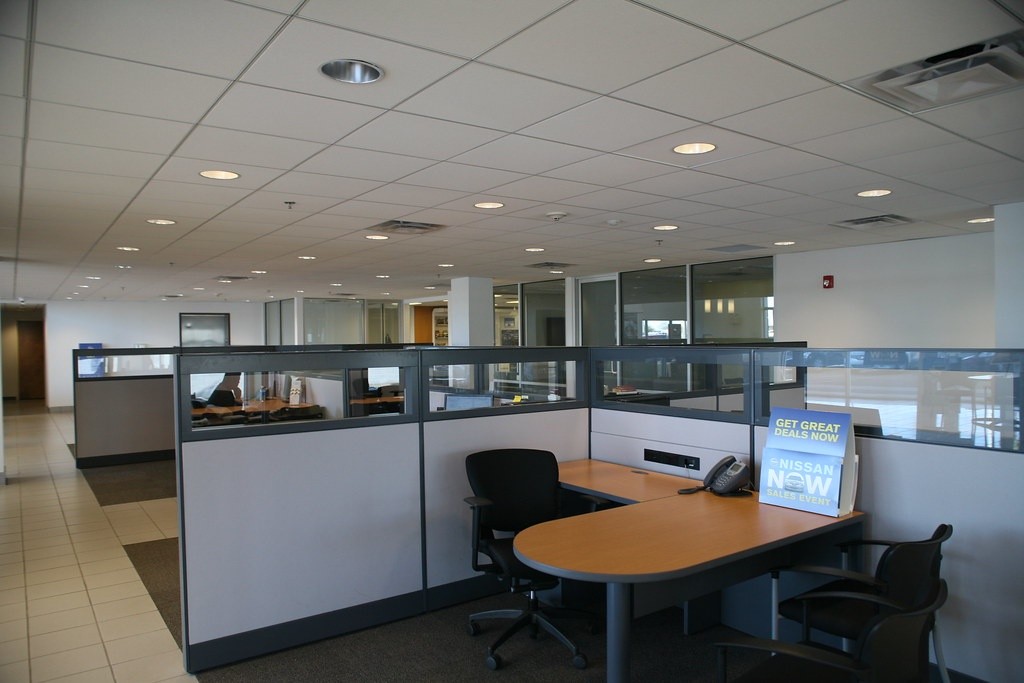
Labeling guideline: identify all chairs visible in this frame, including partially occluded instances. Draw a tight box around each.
[462,449,602,671]
[771,523,954,683]
[352,378,400,414]
[202,410,247,426]
[714,577,948,683]
[191,393,207,409]
[268,404,323,422]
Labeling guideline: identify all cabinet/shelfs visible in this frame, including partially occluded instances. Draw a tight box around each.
[431,308,448,347]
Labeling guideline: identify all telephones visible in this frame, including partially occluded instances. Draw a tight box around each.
[703,456,749,495]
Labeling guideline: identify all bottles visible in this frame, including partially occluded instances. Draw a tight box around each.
[260,386,265,401]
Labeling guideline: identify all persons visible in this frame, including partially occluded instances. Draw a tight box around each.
[208,372,243,407]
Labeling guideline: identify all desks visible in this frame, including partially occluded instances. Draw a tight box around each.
[560,458,721,637]
[351,396,404,416]
[192,400,315,422]
[512,489,865,683]
[247,395,286,405]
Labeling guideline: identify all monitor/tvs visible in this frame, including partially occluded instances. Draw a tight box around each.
[444,393,494,411]
[367,367,400,392]
